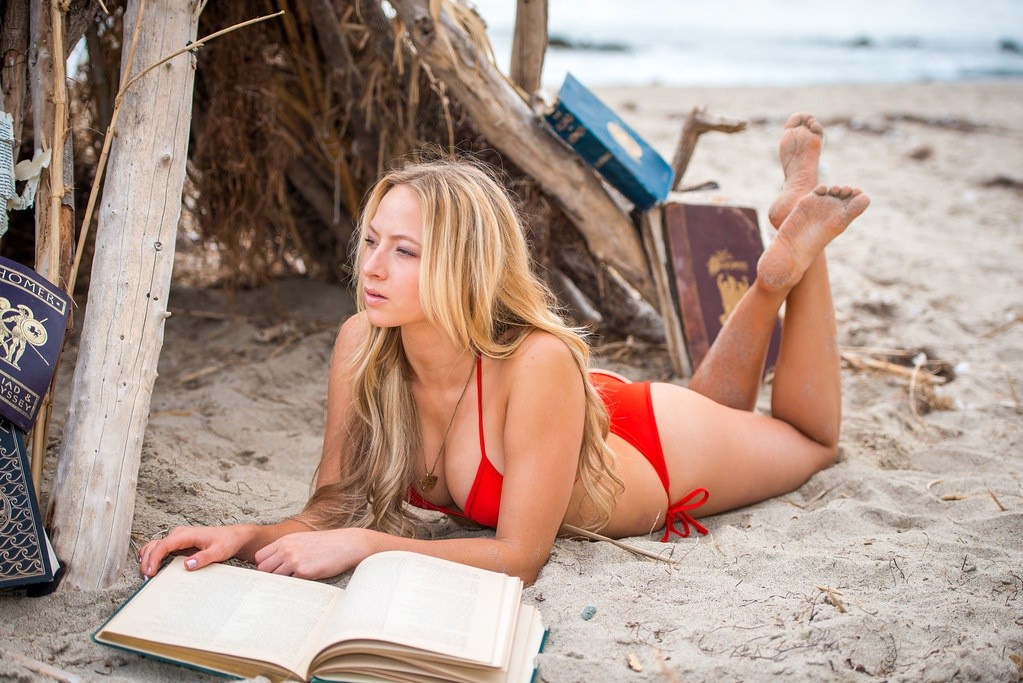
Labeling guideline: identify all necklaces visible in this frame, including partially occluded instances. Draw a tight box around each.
[407,354,476,491]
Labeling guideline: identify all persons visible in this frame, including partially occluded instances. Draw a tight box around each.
[140,114,870,588]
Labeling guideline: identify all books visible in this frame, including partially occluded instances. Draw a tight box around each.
[542,71,782,381]
[0,256,70,585]
[92,551,549,683]
